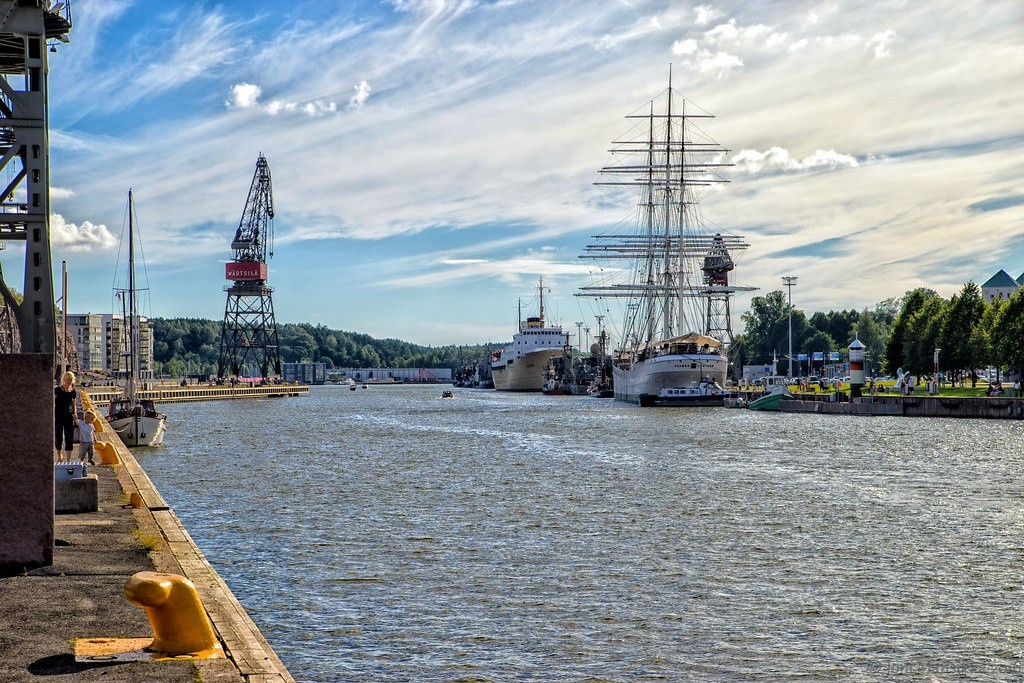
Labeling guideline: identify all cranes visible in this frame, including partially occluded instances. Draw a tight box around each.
[217,151,282,385]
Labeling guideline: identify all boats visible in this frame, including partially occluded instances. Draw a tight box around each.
[638,364,739,408]
[541,330,614,398]
[350,384,356,391]
[747,376,794,411]
[490,274,567,392]
[442,391,454,397]
[723,396,747,409]
[344,378,355,385]
[362,384,368,389]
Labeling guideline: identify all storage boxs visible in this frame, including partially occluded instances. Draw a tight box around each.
[55,460,83,479]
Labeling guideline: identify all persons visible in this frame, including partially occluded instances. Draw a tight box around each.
[75,410,98,466]
[1013,379,1024,397]
[986,383,1002,397]
[54,372,77,462]
[900,378,905,395]
[908,378,914,395]
[819,379,829,391]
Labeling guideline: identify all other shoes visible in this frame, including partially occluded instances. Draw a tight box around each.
[88,459,96,465]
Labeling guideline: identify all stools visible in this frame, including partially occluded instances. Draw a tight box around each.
[55,473,98,513]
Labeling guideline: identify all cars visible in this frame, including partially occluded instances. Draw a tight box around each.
[976,369,1005,384]
[749,377,766,386]
[938,373,947,381]
[775,376,851,387]
[864,376,885,383]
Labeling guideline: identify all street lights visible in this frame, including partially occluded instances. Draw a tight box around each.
[582,328,592,352]
[574,321,583,352]
[595,315,606,336]
[780,276,798,380]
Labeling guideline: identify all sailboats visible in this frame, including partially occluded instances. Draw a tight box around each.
[572,61,762,407]
[103,187,167,448]
